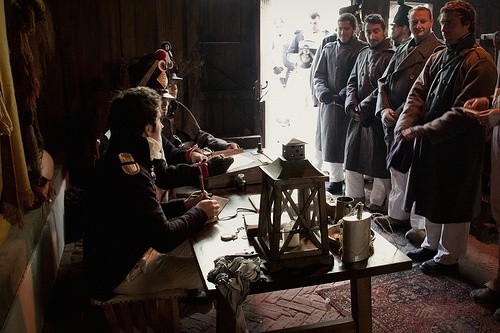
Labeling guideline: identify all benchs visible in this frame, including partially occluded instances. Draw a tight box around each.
[91,289,187,333]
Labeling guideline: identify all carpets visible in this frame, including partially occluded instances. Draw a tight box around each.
[316,262,500,333]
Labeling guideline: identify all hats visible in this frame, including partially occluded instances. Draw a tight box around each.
[160,86,176,99]
[389,4,413,27]
[171,71,183,80]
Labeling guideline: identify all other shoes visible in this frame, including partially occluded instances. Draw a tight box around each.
[407,248,438,261]
[405,228,426,242]
[352,197,365,207]
[470,287,496,300]
[419,259,459,273]
[374,215,409,228]
[327,182,342,195]
[366,203,384,213]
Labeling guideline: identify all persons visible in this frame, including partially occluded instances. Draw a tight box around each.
[273,0,500,321]
[82,47,240,319]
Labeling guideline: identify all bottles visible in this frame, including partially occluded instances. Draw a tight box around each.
[343,203,372,262]
[236,173,247,192]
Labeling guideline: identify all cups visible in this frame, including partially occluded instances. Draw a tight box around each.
[334,197,354,224]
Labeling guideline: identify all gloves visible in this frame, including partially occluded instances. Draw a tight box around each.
[207,154,234,175]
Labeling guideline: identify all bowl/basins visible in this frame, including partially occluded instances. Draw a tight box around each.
[328,224,375,252]
[179,141,195,149]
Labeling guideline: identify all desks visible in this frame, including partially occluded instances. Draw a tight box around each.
[173,147,413,333]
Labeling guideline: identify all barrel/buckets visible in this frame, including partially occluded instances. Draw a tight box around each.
[283,220,300,247]
